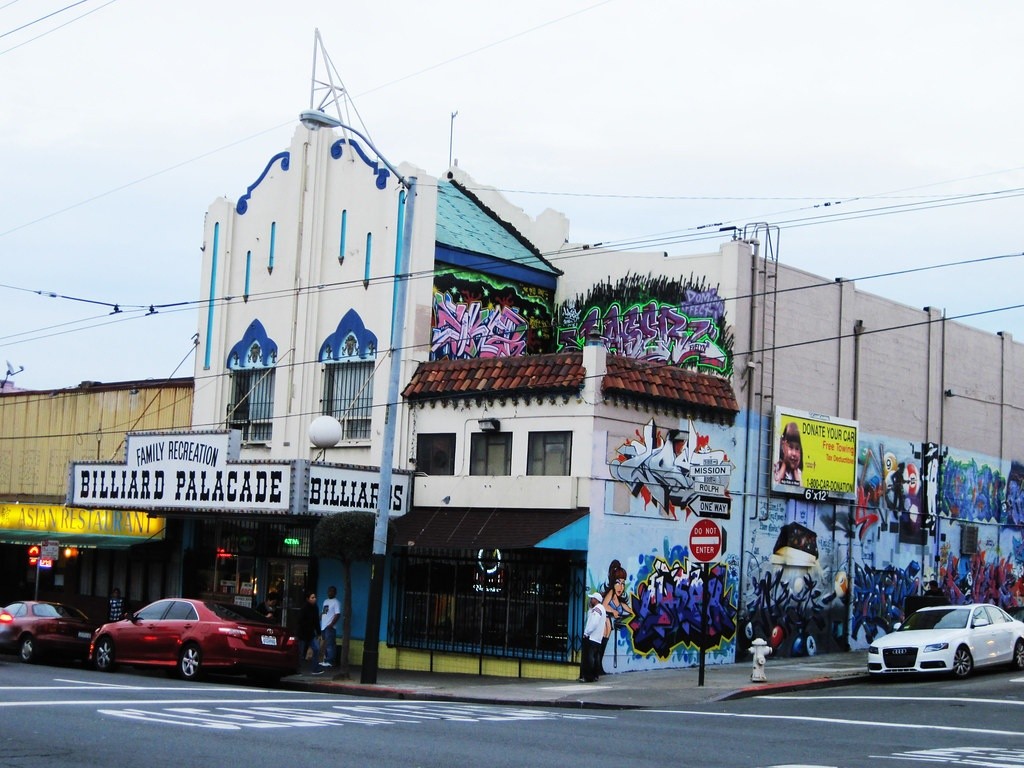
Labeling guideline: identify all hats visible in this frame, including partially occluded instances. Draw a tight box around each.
[588,592,603,602]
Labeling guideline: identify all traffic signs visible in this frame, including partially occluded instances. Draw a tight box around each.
[689,464,731,476]
[686,495,731,521]
[693,480,725,496]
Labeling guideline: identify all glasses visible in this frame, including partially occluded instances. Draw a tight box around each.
[310,596,317,599]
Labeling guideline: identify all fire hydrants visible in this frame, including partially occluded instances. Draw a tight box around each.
[747,637,773,682]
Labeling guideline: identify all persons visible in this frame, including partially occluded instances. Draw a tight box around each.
[775,423,804,485]
[108,588,127,622]
[294,592,325,676]
[257,592,281,624]
[319,586,340,667]
[577,592,606,682]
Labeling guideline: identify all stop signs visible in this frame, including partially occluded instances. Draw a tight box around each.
[689,517,723,563]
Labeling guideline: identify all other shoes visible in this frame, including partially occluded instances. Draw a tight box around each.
[579,677,595,682]
[322,662,333,667]
[318,661,325,666]
[312,670,325,675]
[295,672,303,677]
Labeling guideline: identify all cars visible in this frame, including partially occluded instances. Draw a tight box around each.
[89,598,301,687]
[867,603,1024,681]
[0,599,99,663]
